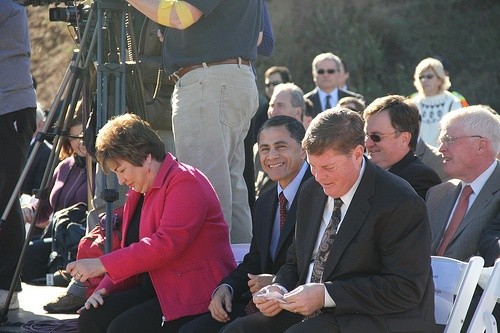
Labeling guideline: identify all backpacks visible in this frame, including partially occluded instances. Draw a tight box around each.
[76,201,132,305]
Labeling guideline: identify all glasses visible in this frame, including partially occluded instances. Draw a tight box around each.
[438,134,487,145]
[264,81,282,88]
[315,69,340,74]
[364,128,407,142]
[417,72,441,80]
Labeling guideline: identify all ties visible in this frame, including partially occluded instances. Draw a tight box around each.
[244,193,288,314]
[434,183,475,260]
[301,194,342,323]
[325,93,332,113]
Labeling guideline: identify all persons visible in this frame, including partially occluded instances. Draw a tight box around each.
[264,66,291,101]
[426,104,499,332]
[415,114,457,185]
[436,54,469,107]
[303,53,365,116]
[257,0,274,57]
[215,107,437,333]
[19,75,100,285]
[1,0,37,312]
[364,95,442,203]
[66,113,237,333]
[44,7,175,313]
[179,116,315,333]
[252,82,307,202]
[126,0,264,244]
[304,98,316,131]
[337,97,367,116]
[405,57,464,154]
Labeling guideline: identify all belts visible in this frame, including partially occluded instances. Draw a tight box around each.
[165,55,254,83]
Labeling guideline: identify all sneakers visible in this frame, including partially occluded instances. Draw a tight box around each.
[0,288,20,311]
[44,290,89,313]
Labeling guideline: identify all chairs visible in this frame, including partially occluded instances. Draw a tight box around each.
[229,242,500,333]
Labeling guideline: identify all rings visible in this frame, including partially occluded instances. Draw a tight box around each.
[294,309,299,315]
